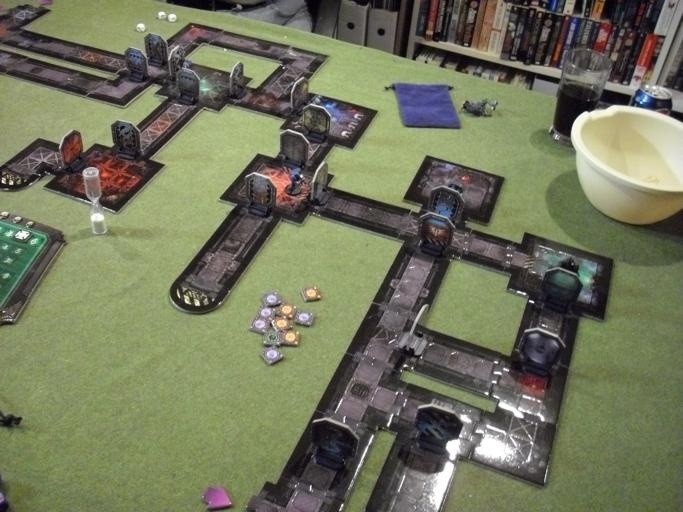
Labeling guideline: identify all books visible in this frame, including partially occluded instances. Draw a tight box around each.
[411,1,683,88]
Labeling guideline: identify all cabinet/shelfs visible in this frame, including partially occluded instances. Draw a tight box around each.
[404,0,683,114]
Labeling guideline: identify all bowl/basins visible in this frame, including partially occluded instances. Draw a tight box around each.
[570,105,683,226]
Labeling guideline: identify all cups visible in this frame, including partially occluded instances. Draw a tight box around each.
[546,48,613,148]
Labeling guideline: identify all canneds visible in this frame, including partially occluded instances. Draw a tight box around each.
[628,83,672,117]
